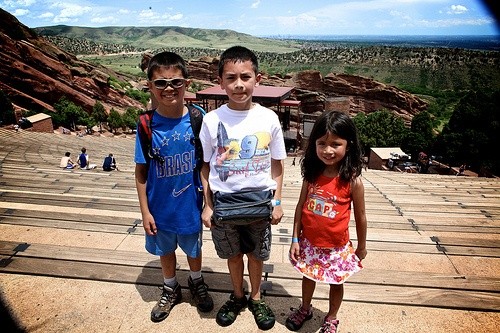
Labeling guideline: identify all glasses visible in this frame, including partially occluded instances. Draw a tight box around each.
[150,78,187,90]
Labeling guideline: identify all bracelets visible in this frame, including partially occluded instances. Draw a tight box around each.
[292,238,299,243]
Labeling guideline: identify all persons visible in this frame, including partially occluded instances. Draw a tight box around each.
[388,151,467,175]
[198,46,287,331]
[297,157,303,166]
[103,153,119,172]
[59,152,77,169]
[285,111,368,332]
[76,148,97,170]
[134,51,213,323]
[290,158,295,167]
[14,123,19,132]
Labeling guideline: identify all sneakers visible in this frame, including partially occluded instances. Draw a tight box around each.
[248,296,275,330]
[285,303,313,331]
[319,316,339,333]
[216,292,247,327]
[151,282,182,322]
[188,275,214,313]
